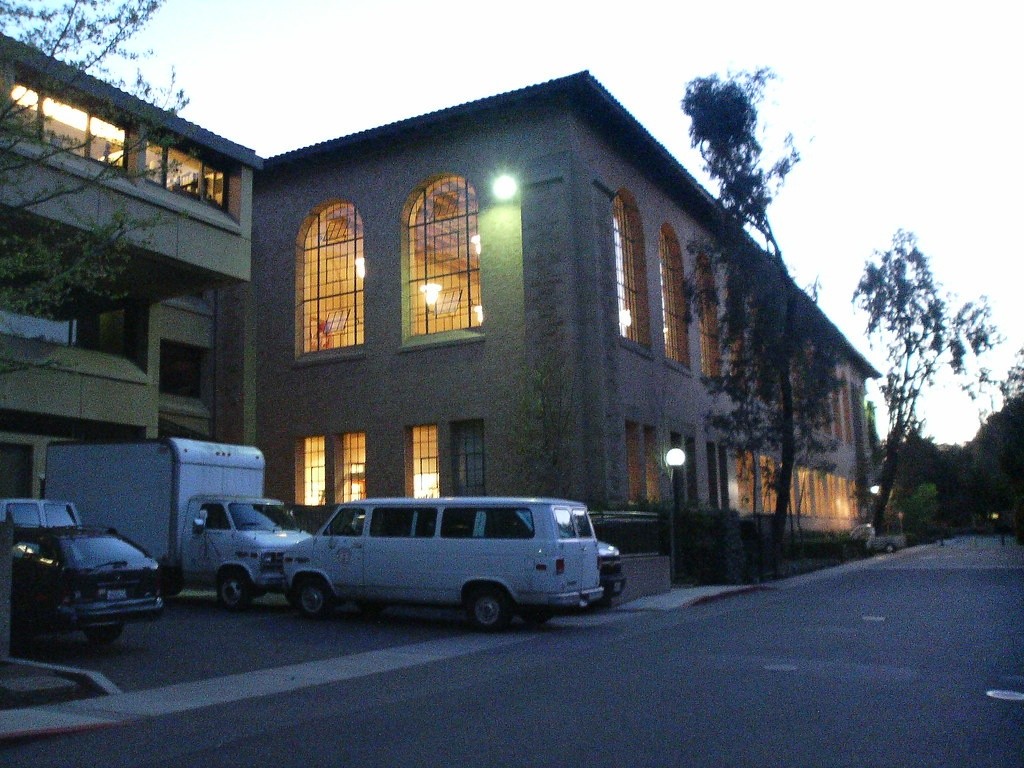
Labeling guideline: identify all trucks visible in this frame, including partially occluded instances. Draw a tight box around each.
[44,437,311,613]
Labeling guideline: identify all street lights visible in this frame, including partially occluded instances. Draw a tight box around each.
[665,448,687,585]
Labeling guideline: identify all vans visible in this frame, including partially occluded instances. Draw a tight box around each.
[282,496,606,630]
[0,497,83,534]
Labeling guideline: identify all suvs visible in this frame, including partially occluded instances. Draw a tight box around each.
[453,506,627,611]
[12,523,166,650]
